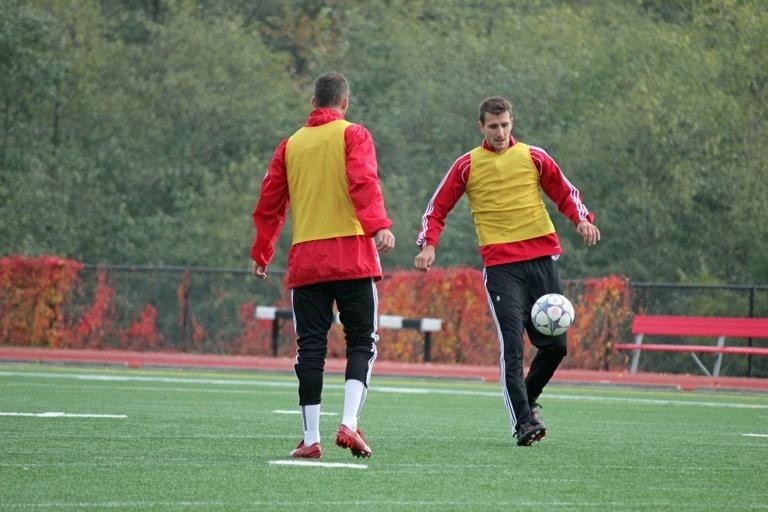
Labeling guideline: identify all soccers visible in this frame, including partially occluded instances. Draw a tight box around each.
[531,293,575,336]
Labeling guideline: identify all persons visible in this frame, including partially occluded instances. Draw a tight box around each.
[249,73,396,459]
[414,95,602,446]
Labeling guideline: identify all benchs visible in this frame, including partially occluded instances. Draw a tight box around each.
[608,310,767,379]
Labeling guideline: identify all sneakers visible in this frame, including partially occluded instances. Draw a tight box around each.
[513,401,547,445]
[290,440,322,458]
[335,423,371,458]
